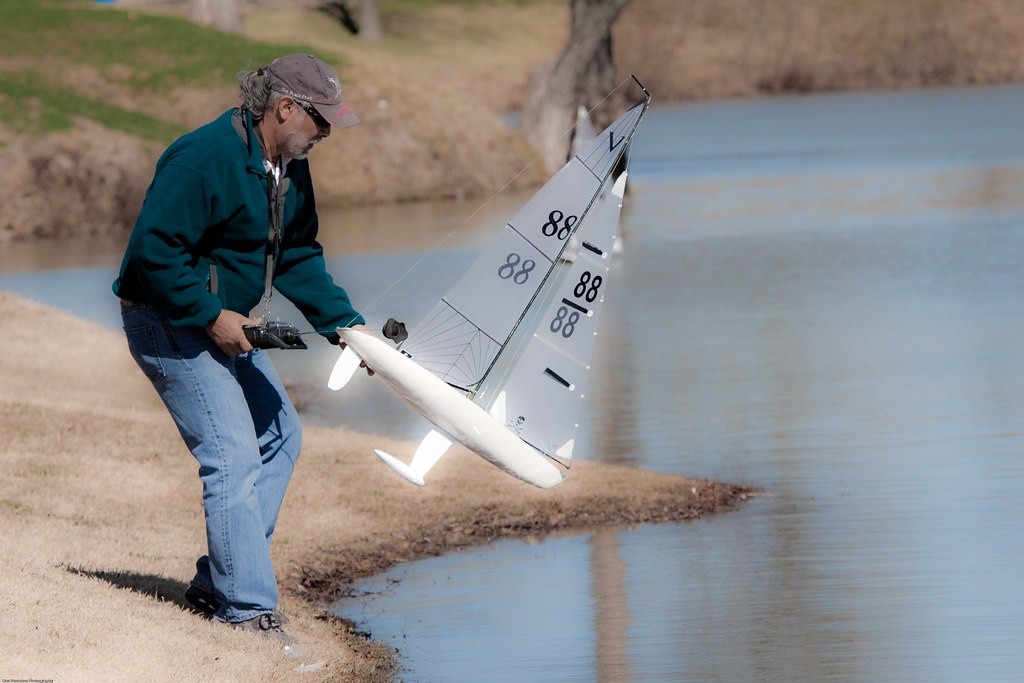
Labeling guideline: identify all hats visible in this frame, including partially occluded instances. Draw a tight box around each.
[264,54,360,128]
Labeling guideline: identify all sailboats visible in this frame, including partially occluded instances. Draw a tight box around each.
[328,73,654,486]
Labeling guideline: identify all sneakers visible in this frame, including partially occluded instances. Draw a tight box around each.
[185,584,290,625]
[213,613,308,658]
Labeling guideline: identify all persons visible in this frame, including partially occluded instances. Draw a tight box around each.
[112,52,378,648]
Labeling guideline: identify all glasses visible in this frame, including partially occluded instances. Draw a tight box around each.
[293,98,331,128]
